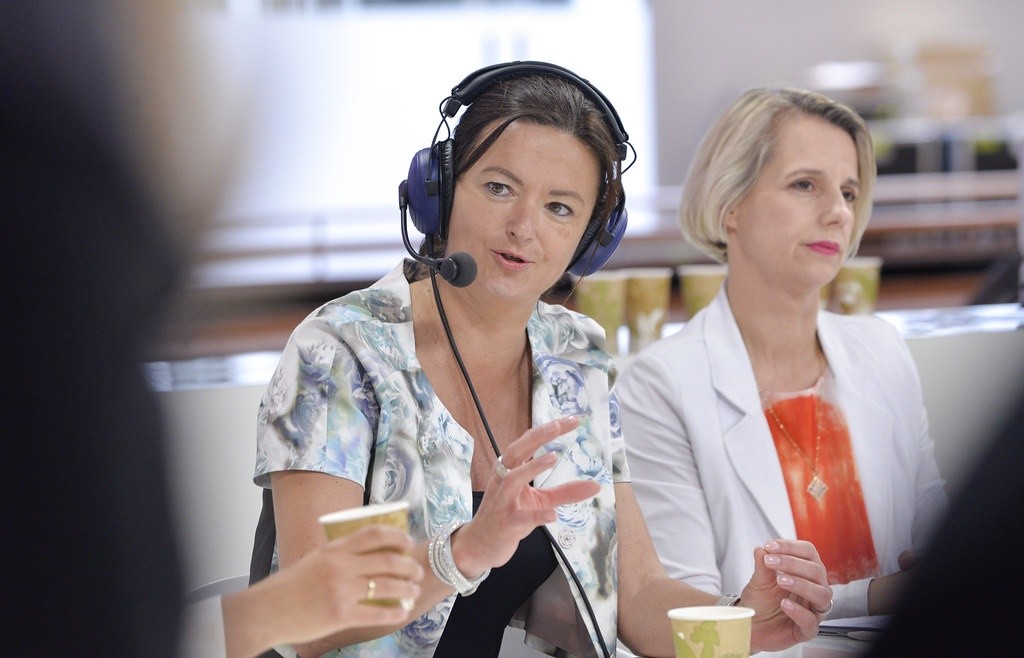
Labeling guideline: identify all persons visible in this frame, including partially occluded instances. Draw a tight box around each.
[187,518,421,655]
[615,84,952,618]
[256,60,836,656]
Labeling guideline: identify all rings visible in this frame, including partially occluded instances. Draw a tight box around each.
[495,455,512,482]
[362,577,378,602]
[808,597,834,616]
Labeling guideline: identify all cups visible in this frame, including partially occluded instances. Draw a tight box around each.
[820,255,885,313]
[319,501,412,611]
[573,270,625,354]
[667,605,755,657]
[621,267,673,353]
[679,265,728,321]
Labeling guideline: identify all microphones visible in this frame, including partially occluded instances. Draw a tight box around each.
[396,181,476,287]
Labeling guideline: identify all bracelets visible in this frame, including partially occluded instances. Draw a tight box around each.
[714,589,744,608]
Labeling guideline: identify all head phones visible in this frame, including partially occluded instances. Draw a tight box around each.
[398,61,638,278]
